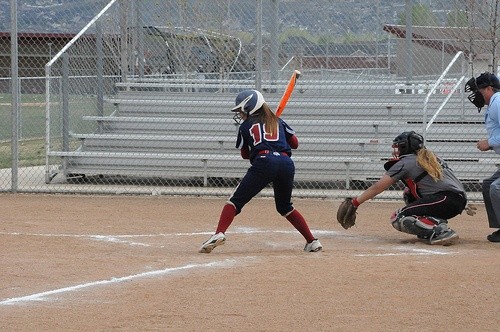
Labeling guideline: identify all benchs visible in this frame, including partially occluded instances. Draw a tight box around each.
[48,71,500,188]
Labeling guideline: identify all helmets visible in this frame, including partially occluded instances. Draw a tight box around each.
[392,131,426,156]
[231,90,265,117]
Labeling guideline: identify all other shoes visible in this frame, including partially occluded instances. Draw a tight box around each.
[487,230,500,242]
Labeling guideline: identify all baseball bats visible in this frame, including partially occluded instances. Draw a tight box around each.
[276,69,302,116]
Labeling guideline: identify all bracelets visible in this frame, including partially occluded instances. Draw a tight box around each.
[352,198,360,208]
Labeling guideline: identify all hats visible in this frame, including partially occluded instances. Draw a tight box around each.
[468,73,500,89]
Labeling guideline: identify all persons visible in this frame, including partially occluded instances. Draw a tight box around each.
[337,130,467,246]
[198,90,323,254]
[464,71,500,242]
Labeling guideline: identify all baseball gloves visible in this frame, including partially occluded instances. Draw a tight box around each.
[336,196,358,231]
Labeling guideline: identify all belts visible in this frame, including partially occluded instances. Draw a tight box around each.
[257,149,288,157]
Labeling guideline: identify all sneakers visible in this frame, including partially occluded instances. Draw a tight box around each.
[198,232,226,254]
[304,239,323,253]
[428,227,460,246]
